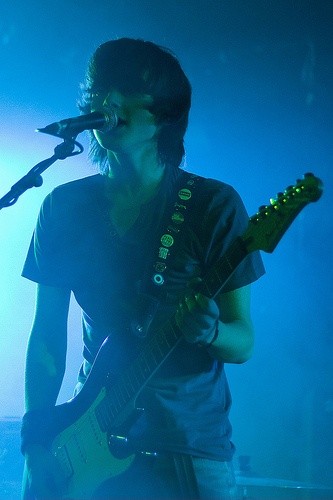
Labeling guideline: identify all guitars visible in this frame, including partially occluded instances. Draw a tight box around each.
[22,171,324,500]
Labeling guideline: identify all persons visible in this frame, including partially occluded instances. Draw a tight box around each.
[21,38,267,500]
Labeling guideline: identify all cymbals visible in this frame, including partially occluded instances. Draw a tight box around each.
[233,471,331,492]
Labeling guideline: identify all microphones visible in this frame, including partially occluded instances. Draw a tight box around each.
[36,106,118,134]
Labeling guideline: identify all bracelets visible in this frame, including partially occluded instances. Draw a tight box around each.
[205,319,220,346]
